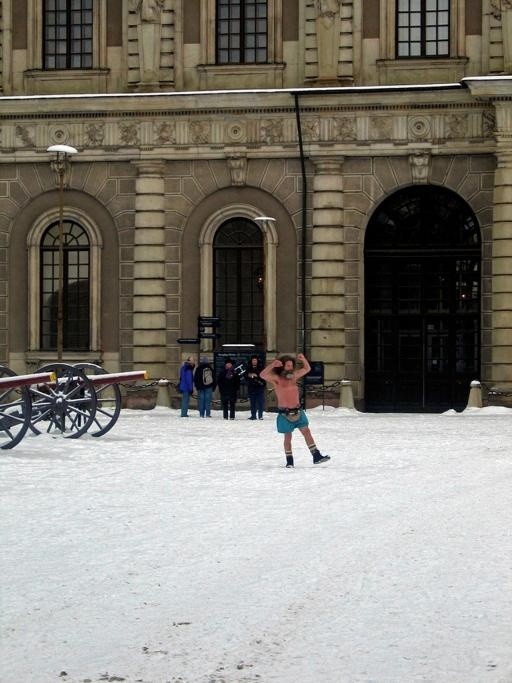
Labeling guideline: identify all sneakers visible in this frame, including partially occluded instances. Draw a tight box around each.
[248,415,263,420]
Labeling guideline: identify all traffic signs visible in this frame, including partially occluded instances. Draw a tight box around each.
[177,338,201,344]
[198,332,221,338]
[198,316,220,322]
[198,322,220,327]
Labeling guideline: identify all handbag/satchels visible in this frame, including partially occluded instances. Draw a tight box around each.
[203,368,212,386]
[280,408,300,422]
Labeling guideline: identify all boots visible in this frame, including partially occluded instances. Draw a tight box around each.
[312,450,330,463]
[286,455,294,468]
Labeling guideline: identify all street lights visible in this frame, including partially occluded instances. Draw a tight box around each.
[47,142,78,368]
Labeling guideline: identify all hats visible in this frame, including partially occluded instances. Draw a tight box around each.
[202,356,209,363]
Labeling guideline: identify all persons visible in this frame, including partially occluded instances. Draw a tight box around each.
[243,355,268,421]
[178,356,196,417]
[261,351,332,468]
[194,355,218,417]
[218,358,241,420]
